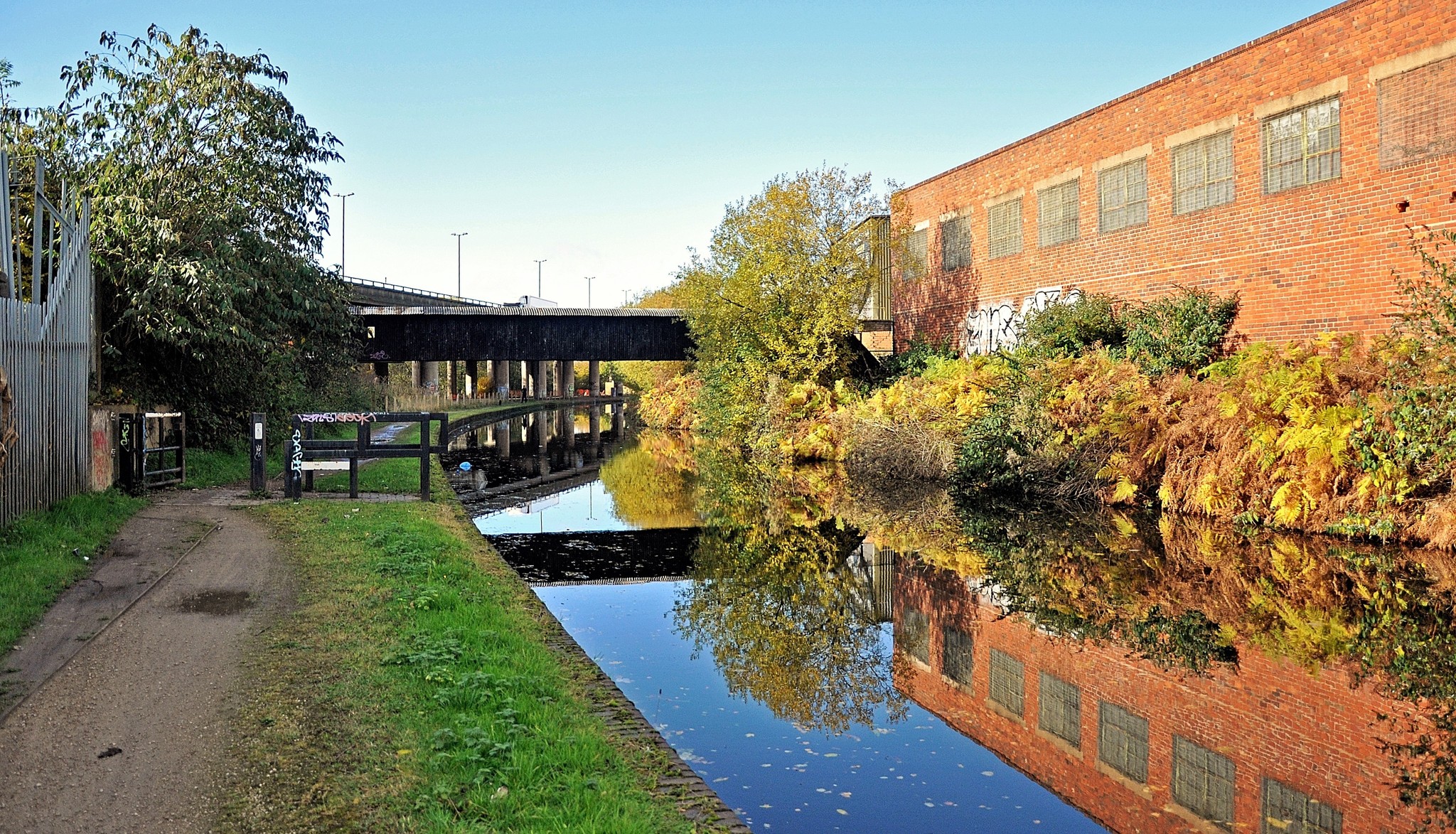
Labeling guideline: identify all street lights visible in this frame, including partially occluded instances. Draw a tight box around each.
[584,276,595,308]
[451,233,469,297]
[622,289,631,304]
[534,260,546,297]
[333,192,354,281]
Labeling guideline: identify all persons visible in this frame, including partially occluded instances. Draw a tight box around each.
[521,385,527,403]
[521,414,527,429]
